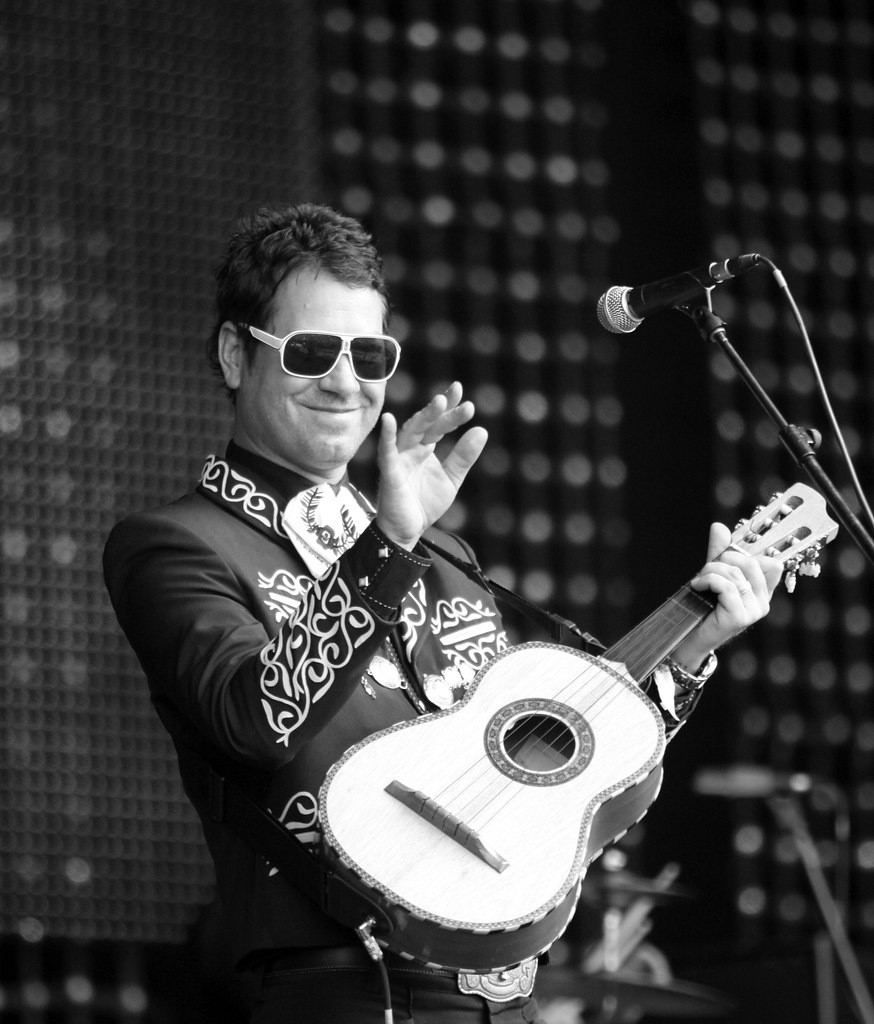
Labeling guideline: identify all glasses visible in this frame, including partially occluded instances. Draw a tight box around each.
[247,329,403,383]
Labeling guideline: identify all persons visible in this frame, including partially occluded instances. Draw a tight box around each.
[102,205,787,1024]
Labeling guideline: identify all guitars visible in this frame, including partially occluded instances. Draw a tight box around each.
[315,480,841,978]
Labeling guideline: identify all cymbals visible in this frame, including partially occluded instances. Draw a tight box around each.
[541,961,738,1011]
[581,867,692,902]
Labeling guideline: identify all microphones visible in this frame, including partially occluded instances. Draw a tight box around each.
[694,763,808,797]
[598,253,760,333]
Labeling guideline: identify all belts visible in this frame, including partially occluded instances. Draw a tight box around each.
[245,945,539,1002]
